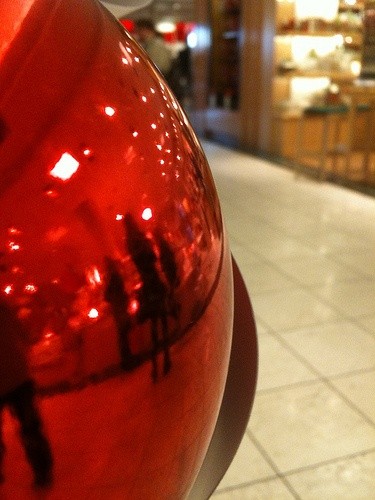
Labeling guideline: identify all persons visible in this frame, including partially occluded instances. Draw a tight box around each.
[131,17,174,78]
